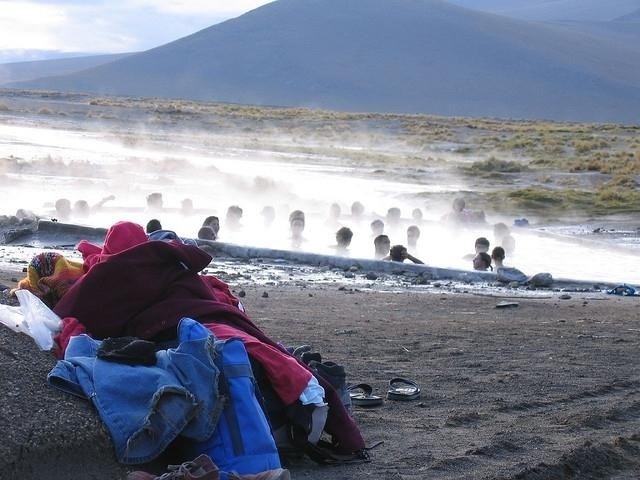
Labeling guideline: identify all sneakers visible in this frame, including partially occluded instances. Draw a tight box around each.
[127,454,220,479]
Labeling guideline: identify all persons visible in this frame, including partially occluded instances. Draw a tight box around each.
[29,162,521,274]
[52,218,373,469]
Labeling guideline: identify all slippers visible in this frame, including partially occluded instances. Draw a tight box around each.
[387,377,421,401]
[347,383,384,407]
